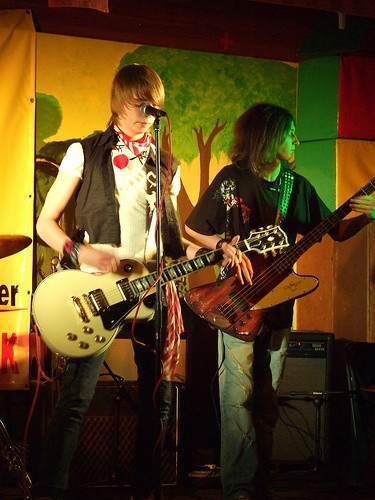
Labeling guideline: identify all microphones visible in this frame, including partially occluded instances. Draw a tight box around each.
[140,103,167,117]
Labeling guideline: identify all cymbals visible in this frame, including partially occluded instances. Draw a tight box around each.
[0,234,32,260]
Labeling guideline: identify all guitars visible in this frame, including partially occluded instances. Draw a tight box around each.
[184,177,375,340]
[33,224,292,359]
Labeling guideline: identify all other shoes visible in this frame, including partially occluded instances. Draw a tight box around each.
[236,491,253,500]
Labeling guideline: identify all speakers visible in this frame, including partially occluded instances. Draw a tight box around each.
[266,330,333,465]
[331,341,375,487]
[48,380,179,488]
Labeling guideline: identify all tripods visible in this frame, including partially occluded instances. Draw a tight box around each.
[74,360,159,489]
[275,388,363,480]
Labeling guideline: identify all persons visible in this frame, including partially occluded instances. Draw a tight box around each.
[30,64,242,500]
[184,101,375,500]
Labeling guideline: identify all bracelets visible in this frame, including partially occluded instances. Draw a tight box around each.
[215,238,229,250]
[59,240,83,268]
